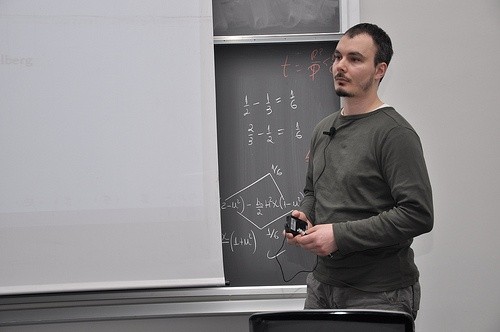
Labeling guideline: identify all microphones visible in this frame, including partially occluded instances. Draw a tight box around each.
[323,127,336,136]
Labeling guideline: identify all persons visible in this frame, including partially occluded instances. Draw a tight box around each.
[283,23,434,320]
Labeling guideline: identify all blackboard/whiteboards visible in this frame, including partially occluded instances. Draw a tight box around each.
[212,0,343,36]
[0,31,344,313]
